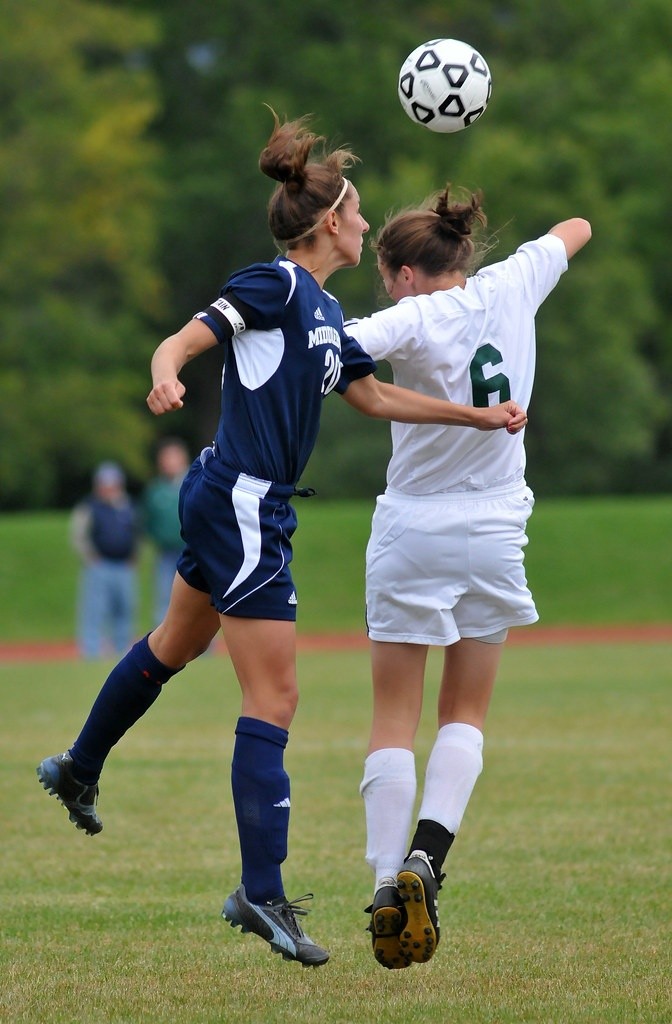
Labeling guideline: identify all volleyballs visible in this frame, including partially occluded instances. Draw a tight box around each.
[399,38,493,133]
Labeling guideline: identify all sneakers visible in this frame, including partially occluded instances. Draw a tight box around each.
[365,848,441,969]
[220,886,331,967]
[34,753,104,835]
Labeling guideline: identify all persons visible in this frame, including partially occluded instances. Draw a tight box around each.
[141,443,215,659]
[69,459,137,660]
[340,183,591,970]
[35,102,525,970]
